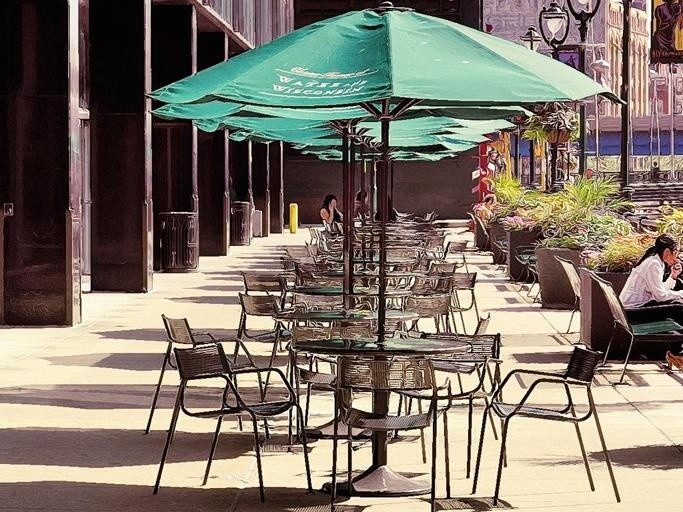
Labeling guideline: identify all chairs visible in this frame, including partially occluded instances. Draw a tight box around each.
[329,355,452,510]
[554,254,582,333]
[410,333,508,466]
[145,315,267,434]
[514,245,539,286]
[472,347,620,504]
[153,344,315,502]
[588,270,683,385]
[492,238,509,269]
[231,293,314,392]
[527,262,540,302]
[238,211,481,334]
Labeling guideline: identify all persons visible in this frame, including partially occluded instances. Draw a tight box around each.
[475,194,497,220]
[319,194,344,224]
[354,190,369,218]
[619,232,683,373]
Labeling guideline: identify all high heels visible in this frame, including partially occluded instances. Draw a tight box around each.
[666,350,683,370]
